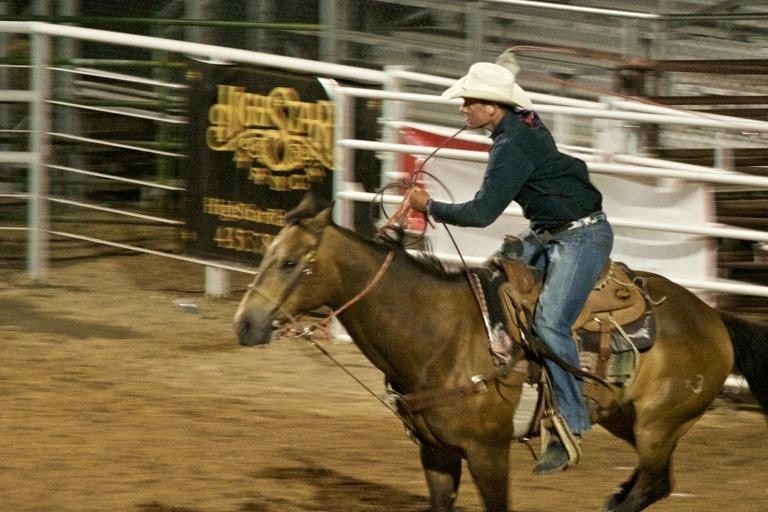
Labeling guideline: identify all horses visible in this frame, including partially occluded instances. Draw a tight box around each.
[226,190,768,512]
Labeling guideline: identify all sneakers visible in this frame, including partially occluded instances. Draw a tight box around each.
[531,435,582,476]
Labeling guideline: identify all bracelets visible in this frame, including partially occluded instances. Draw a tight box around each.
[426,198,432,215]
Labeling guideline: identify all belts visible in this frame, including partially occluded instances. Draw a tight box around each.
[547,208,608,236]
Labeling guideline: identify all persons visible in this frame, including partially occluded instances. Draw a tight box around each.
[408,62,612,474]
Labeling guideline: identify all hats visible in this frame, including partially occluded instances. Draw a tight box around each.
[439,61,535,113]
